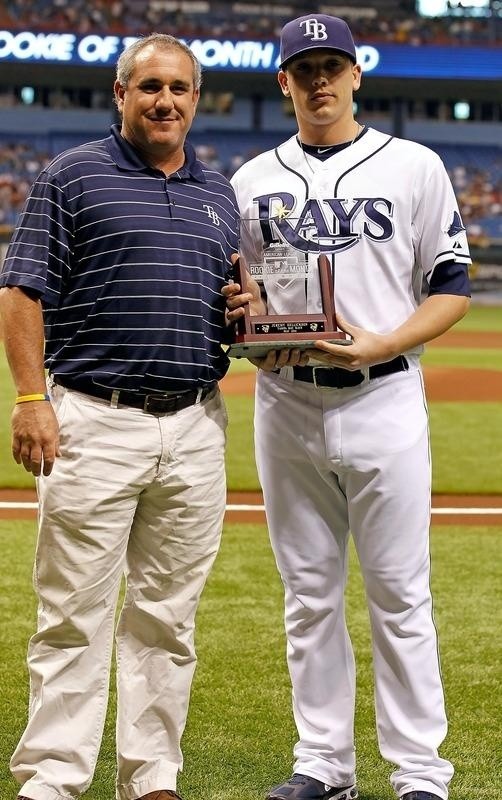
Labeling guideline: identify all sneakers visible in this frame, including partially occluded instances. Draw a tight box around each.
[266,772,359,800]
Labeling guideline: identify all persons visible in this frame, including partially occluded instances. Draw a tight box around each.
[2,30,247,800]
[0,0,500,251]
[219,12,474,798]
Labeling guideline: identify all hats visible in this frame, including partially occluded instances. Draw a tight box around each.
[275,12,357,69]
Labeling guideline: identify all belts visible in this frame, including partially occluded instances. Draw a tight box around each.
[274,355,408,387]
[53,377,213,415]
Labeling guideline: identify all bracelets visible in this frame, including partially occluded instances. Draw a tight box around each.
[16,393,51,404]
